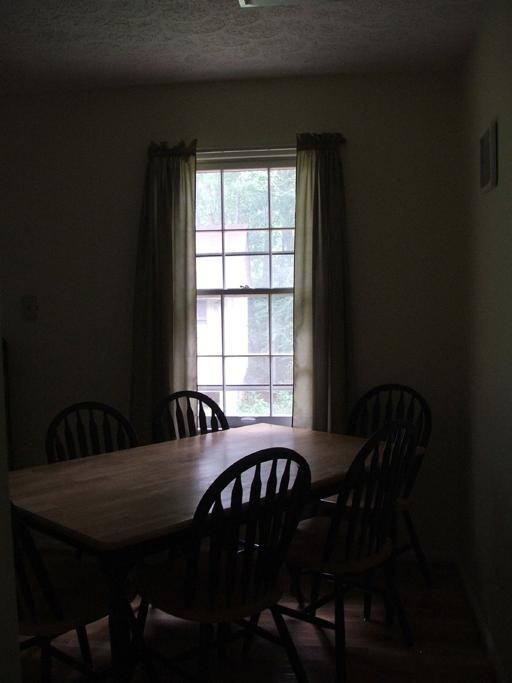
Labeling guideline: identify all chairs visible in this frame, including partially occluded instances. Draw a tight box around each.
[8,499,156,683]
[44,401,139,464]
[151,391,229,443]
[290,420,412,683]
[318,382,430,585]
[128,449,311,683]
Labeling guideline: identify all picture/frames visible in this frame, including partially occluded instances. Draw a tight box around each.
[476,125,497,192]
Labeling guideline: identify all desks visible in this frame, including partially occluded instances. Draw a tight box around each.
[8,425,427,683]
